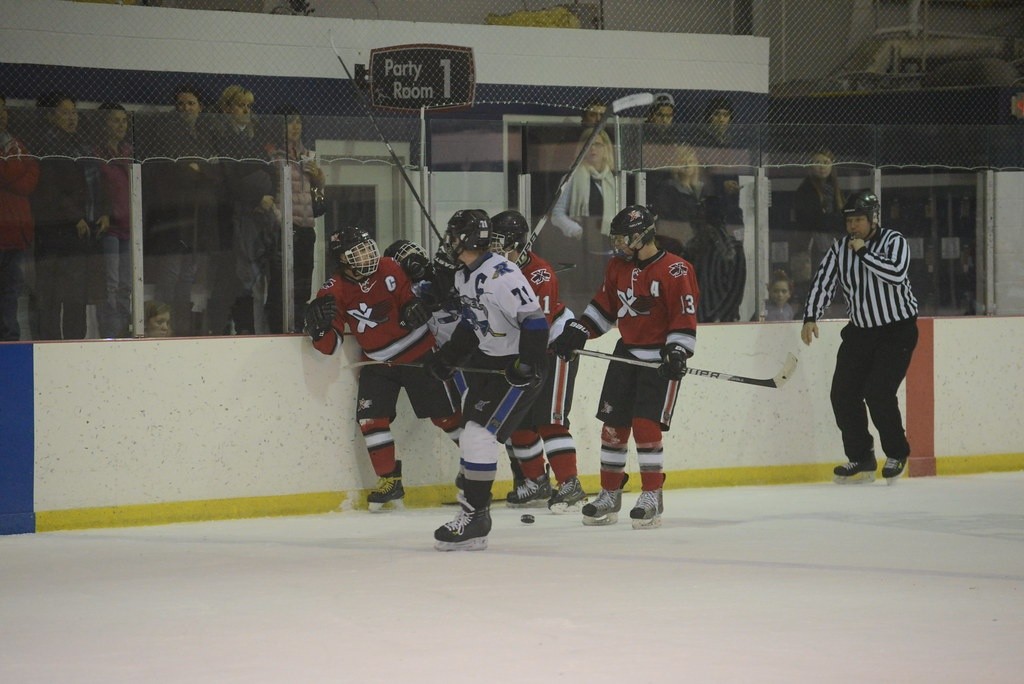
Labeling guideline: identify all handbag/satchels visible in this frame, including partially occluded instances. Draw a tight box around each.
[308,151,327,217]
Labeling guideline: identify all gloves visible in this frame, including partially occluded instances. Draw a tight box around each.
[658,343,687,382]
[548,318,591,363]
[503,358,537,388]
[303,294,338,339]
[422,343,459,384]
[398,298,434,329]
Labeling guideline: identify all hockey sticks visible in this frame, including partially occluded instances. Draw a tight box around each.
[516,92,653,265]
[343,358,506,376]
[326,28,443,241]
[573,347,800,391]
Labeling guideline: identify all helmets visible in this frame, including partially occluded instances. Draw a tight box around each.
[329,227,380,275]
[841,189,878,217]
[491,210,529,255]
[448,209,492,250]
[384,239,429,281]
[610,205,656,263]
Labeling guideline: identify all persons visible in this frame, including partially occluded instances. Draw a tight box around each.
[144,300,174,337]
[29,94,91,340]
[93,102,145,338]
[550,91,976,323]
[0,95,39,341]
[767,275,794,321]
[548,204,700,529]
[801,190,918,487]
[305,225,476,515]
[396,208,549,552]
[154,85,325,336]
[488,210,588,514]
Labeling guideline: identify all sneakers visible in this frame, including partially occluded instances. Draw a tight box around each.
[630,474,666,530]
[367,459,405,513]
[506,463,552,508]
[881,454,906,486]
[548,474,588,514]
[582,473,629,526]
[434,492,492,550]
[833,441,877,484]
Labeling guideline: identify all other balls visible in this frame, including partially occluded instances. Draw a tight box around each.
[521,515,535,523]
